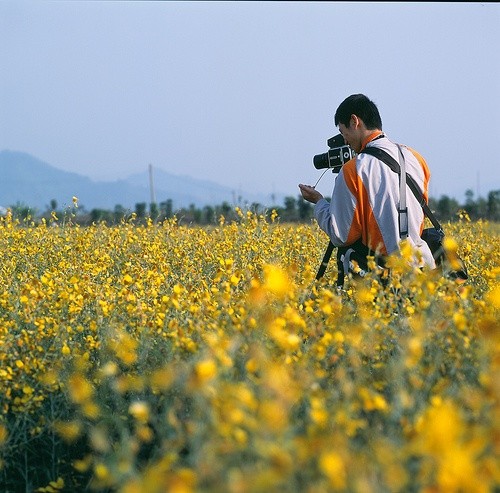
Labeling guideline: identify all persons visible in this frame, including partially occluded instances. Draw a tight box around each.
[298,94,468,286]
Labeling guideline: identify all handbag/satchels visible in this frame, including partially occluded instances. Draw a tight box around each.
[336,225,468,290]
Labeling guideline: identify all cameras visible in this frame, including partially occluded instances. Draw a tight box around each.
[313,134,356,171]
